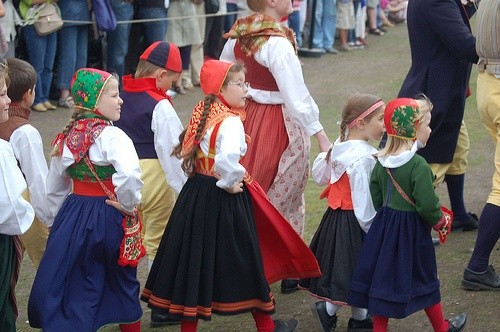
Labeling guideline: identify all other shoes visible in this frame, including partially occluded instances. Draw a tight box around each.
[321,13,405,54]
[31,103,46,112]
[58,96,75,107]
[43,101,57,110]
[174,86,186,94]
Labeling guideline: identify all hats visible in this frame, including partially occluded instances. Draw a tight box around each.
[140,42,182,73]
[200,60,233,95]
[71,68,112,111]
[384,98,420,139]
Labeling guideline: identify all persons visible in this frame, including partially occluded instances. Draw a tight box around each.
[140,58,321,332]
[0,55,56,332]
[298,94,385,332]
[348,94,468,332]
[461,0,500,291]
[377,0,481,231]
[0,0,411,112]
[27,68,146,332]
[113,40,192,327]
[220,0,335,294]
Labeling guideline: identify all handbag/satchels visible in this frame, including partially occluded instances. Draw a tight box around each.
[438,206,453,244]
[34,2,62,36]
[118,213,147,267]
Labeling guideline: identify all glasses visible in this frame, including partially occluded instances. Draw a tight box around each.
[222,82,248,88]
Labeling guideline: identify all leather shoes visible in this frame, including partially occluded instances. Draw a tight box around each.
[451,216,480,231]
[446,314,467,332]
[311,300,338,332]
[271,319,299,332]
[348,316,373,332]
[461,265,500,291]
[432,238,440,245]
[150,309,181,327]
[281,279,300,294]
